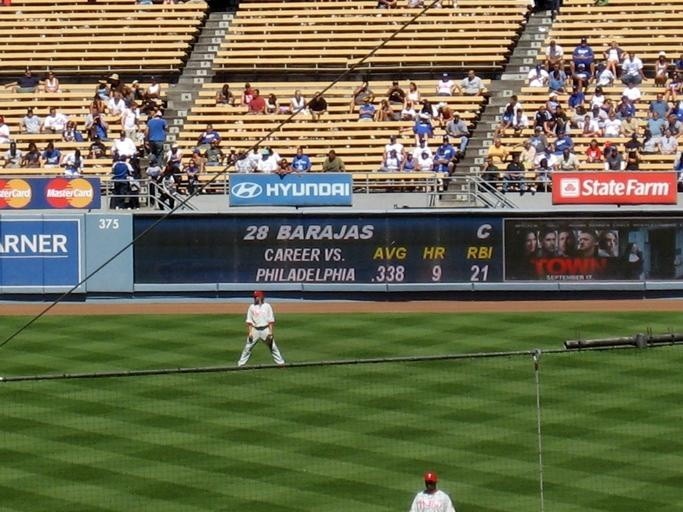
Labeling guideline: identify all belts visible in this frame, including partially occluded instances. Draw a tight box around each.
[255,326,268,330]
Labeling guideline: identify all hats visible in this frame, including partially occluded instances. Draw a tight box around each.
[425,473,437,482]
[252,292,263,297]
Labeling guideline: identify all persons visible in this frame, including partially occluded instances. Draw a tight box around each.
[481,37,682,192]
[409,469,456,512]
[216,81,345,178]
[1,65,222,211]
[350,69,485,190]
[520,229,645,281]
[137,0,175,12]
[237,290,289,368]
[377,0,424,17]
